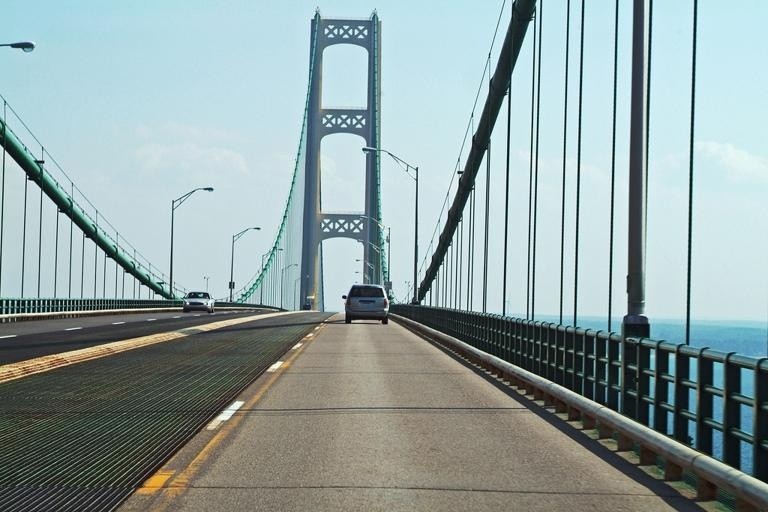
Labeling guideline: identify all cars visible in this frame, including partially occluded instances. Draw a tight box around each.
[182,293,215,313]
[343,285,393,324]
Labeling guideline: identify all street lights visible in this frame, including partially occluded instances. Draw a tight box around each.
[0,43,36,53]
[294,278,301,306]
[281,264,295,310]
[353,239,382,284]
[231,227,259,304]
[359,216,392,298]
[168,187,214,296]
[260,248,281,307]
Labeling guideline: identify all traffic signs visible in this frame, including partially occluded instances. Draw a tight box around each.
[362,147,419,307]
